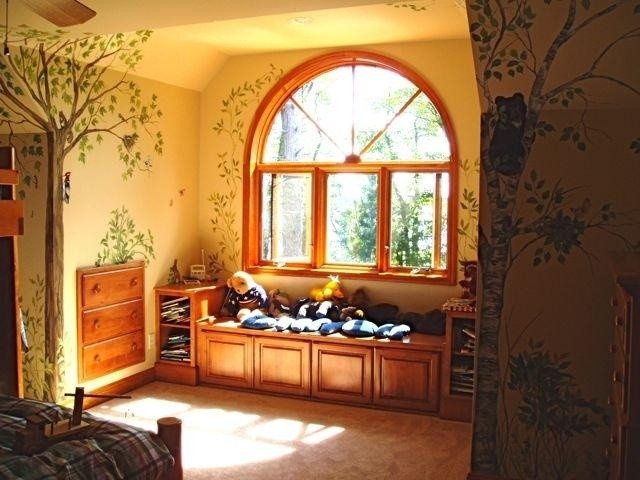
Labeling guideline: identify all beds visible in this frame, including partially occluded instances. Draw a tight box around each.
[1,392,182,479]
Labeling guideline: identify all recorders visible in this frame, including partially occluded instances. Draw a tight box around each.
[190,264,206,280]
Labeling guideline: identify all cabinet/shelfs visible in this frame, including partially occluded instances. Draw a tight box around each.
[154,281,227,386]
[197,316,443,416]
[441,311,475,423]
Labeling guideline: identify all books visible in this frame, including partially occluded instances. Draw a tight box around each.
[449,318,477,395]
[156,295,192,364]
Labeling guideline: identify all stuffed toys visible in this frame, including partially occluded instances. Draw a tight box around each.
[220,270,369,322]
